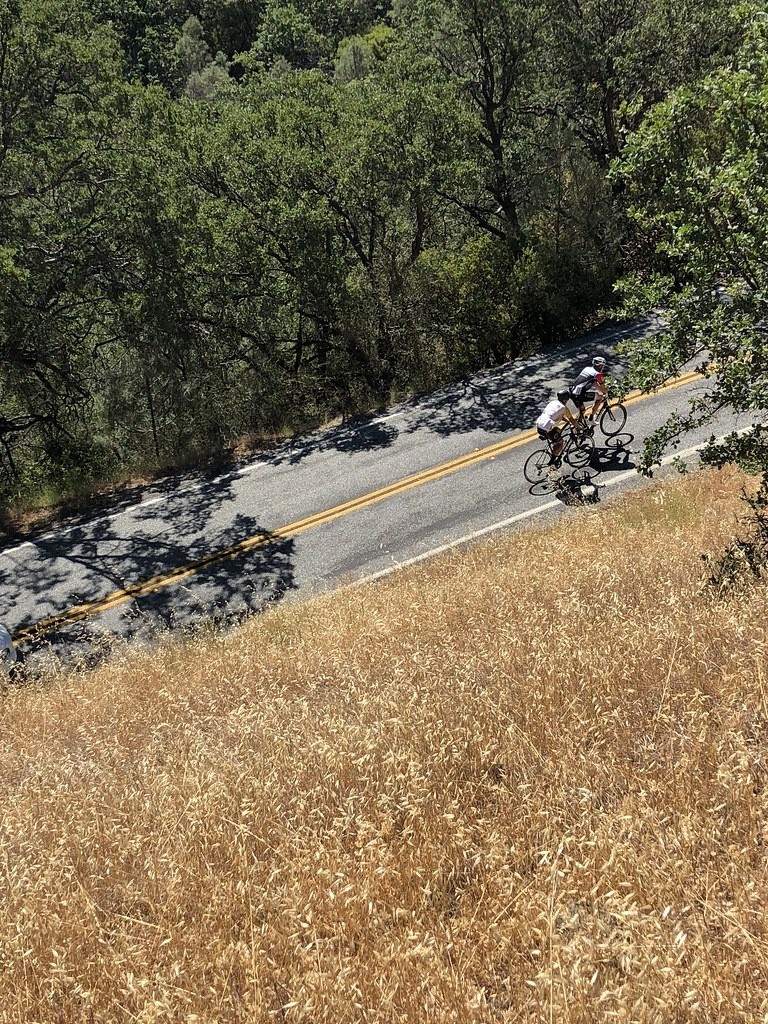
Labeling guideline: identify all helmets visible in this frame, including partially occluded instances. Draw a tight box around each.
[557,390,571,398]
[592,357,606,366]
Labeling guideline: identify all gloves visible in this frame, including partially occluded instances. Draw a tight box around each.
[608,391,613,397]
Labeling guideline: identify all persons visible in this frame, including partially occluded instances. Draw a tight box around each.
[569,357,611,425]
[536,390,581,466]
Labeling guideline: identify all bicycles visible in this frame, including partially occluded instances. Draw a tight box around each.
[524,419,596,485]
[561,388,627,453]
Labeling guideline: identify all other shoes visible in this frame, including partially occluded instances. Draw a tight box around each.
[548,462,559,467]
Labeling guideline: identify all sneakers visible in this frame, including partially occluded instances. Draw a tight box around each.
[586,420,600,425]
